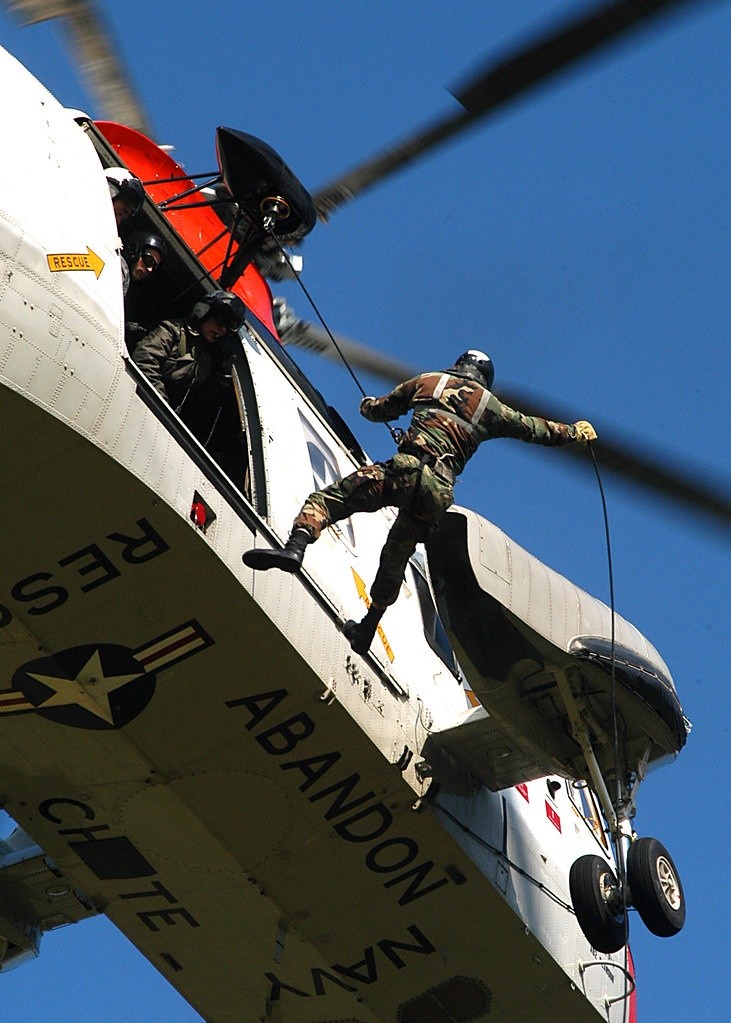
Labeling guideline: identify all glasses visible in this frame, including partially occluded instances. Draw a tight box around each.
[141,251,158,271]
[210,305,237,331]
[119,188,140,210]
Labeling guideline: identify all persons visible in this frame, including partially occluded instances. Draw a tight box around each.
[104,167,145,225]
[242,349,598,653]
[132,291,247,402]
[120,232,166,344]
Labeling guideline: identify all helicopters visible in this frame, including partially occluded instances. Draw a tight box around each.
[0,1,731,1023]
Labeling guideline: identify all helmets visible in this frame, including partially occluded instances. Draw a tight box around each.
[195,290,245,332]
[122,232,168,266]
[455,349,495,390]
[104,166,145,213]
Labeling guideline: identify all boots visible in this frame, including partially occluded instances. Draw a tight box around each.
[343,605,386,654]
[242,530,309,574]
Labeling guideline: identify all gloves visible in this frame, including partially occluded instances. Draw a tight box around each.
[573,420,597,442]
[125,322,146,335]
